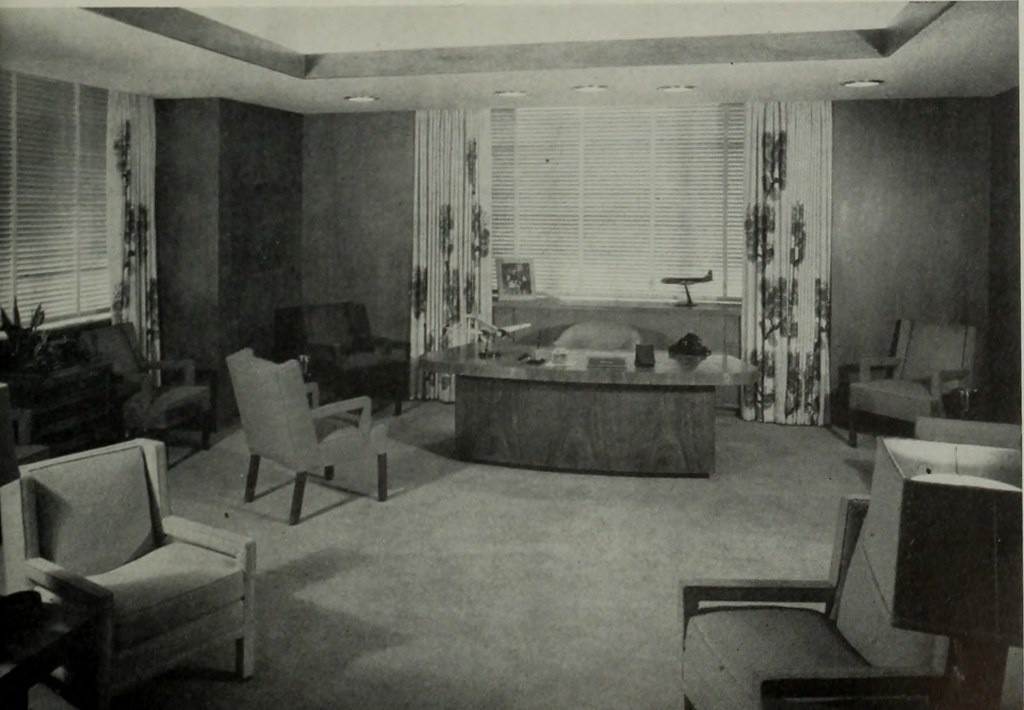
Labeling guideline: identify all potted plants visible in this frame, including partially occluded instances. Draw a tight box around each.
[0,296,69,378]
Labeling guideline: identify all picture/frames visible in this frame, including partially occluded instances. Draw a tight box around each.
[495,256,537,301]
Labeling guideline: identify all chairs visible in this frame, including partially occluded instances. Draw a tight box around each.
[848,317,977,448]
[676,434,1023,708]
[552,322,641,351]
[227,347,390,526]
[0,382,50,466]
[265,301,412,419]
[1,437,258,710]
[70,319,213,471]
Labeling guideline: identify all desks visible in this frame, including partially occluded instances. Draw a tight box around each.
[5,360,113,458]
[418,342,759,475]
